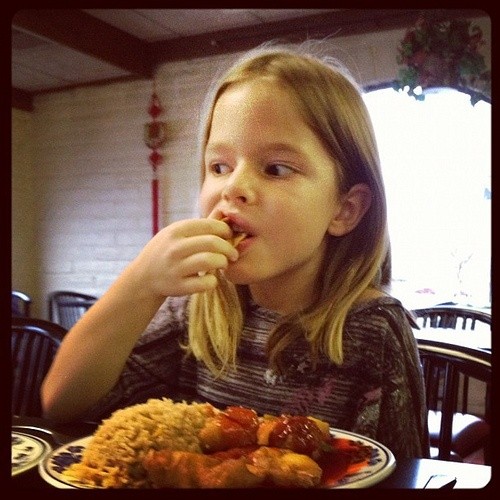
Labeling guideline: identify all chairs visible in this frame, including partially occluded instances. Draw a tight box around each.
[8,290,493,465]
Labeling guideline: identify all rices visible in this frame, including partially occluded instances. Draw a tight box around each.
[63,398,226,487]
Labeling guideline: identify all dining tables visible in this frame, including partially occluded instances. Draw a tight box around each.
[17,434,492,491]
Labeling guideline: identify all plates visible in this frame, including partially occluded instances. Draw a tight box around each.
[38,426,397,489]
[11,424,55,476]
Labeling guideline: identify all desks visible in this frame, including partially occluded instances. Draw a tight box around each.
[411,327,494,352]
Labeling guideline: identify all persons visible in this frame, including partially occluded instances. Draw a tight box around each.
[41,47,431,461]
